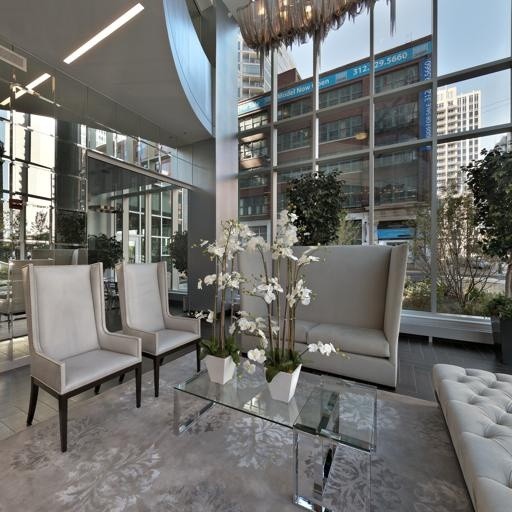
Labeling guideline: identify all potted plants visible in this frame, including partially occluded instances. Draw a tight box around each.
[459,140,512,366]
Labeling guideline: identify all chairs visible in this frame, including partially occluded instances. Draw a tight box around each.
[1,257,54,338]
[103,280,120,316]
[19,259,204,454]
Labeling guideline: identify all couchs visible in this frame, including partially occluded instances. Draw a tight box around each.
[235,243,409,392]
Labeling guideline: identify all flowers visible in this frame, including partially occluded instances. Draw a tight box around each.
[187,208,351,383]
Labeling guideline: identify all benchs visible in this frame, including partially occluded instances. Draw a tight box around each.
[430,362,512,510]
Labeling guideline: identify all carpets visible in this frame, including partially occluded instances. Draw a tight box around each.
[0,347,472,511]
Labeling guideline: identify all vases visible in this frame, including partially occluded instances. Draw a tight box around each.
[205,354,305,405]
